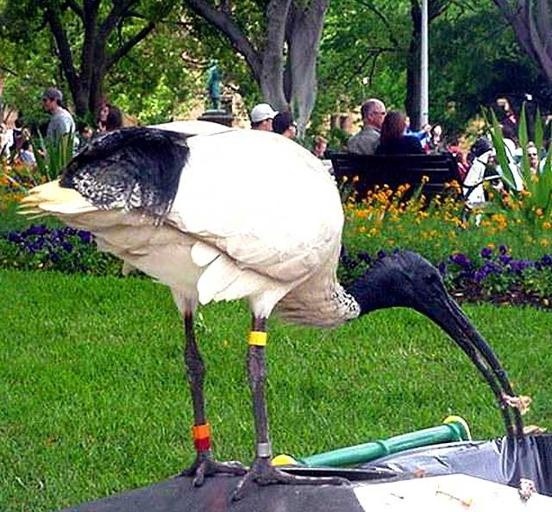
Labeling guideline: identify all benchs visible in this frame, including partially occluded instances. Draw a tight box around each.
[332,151,466,203]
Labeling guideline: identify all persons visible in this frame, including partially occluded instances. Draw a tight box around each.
[0,108,23,166]
[272,110,298,141]
[7,141,37,191]
[35,85,80,161]
[72,120,91,158]
[312,135,329,160]
[347,93,552,228]
[250,101,279,131]
[12,120,31,152]
[95,101,122,132]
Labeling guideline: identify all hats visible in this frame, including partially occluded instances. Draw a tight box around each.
[273,111,293,131]
[251,102,279,122]
[43,88,63,101]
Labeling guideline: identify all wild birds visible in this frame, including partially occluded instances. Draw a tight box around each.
[15,120,525,507]
